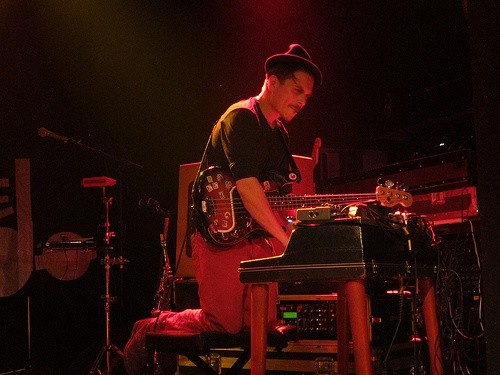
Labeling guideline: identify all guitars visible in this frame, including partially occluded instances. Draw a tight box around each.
[192,166,413,248]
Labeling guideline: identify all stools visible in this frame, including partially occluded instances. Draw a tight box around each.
[150,329,253,375]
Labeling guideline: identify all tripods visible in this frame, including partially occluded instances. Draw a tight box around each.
[89,185,125,375]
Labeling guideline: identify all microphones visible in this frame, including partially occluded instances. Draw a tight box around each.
[38,128,69,143]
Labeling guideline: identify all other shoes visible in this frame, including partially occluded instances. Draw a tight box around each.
[155,349,179,375]
[124,318,153,375]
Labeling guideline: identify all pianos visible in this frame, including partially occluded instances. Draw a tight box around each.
[238,217,443,283]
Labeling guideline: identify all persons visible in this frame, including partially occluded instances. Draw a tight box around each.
[125,45,324,375]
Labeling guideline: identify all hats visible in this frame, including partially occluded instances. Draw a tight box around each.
[264,43,322,88]
